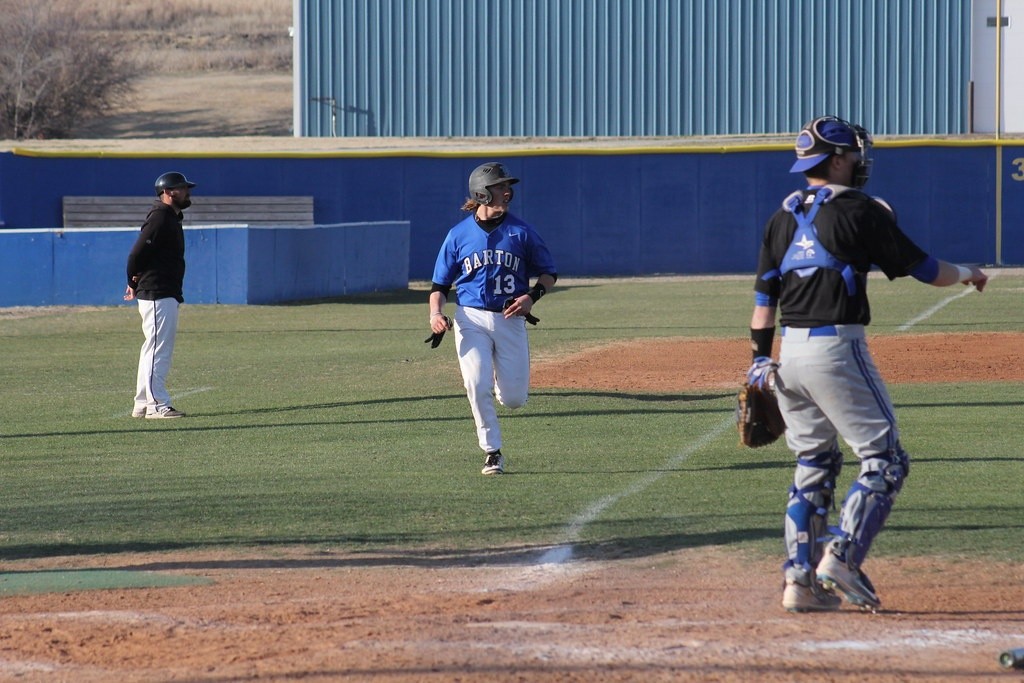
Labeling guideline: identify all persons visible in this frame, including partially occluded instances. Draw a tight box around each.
[124,171,197,418]
[428,162,557,475]
[738,115,987,613]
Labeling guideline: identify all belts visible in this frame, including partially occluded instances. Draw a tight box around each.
[780,326,839,337]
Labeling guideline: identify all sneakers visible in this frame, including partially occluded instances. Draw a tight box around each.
[781,568,843,613]
[482,449,505,475]
[132,406,147,418]
[145,407,185,418]
[816,545,883,609]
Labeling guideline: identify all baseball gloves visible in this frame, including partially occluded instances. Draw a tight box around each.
[736,378,785,449]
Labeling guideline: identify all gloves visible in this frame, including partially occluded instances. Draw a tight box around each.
[425,315,454,349]
[502,296,540,325]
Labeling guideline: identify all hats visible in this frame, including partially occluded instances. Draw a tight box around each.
[789,116,861,172]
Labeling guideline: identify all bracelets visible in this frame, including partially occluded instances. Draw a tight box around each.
[527,283,547,303]
[955,265,972,283]
[430,312,442,323]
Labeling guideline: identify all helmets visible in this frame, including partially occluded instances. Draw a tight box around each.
[154,172,196,196]
[469,162,521,205]
